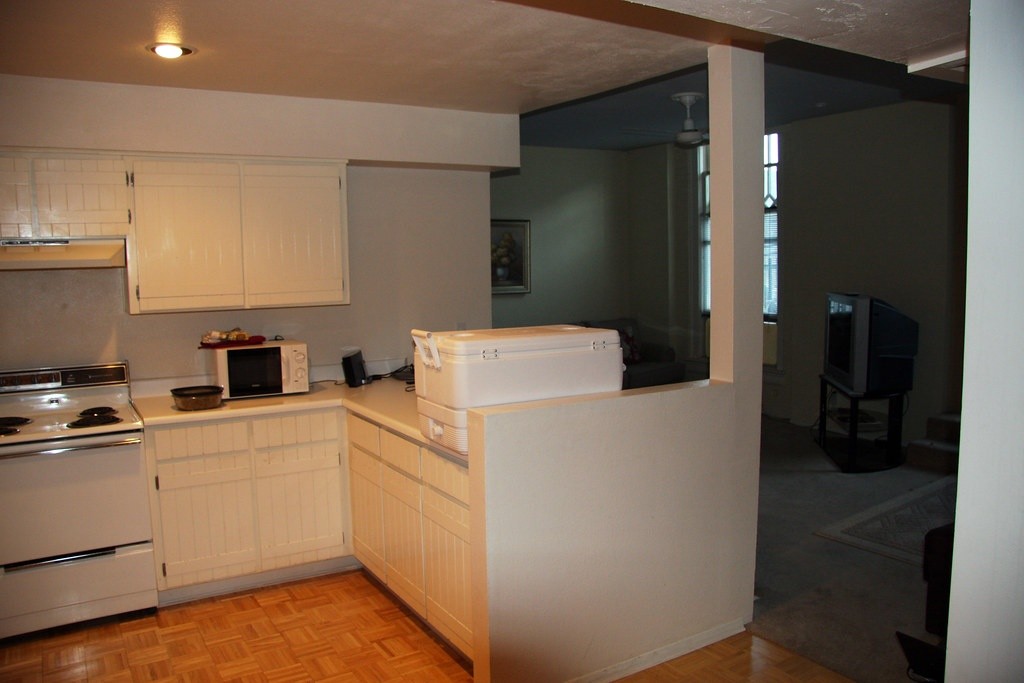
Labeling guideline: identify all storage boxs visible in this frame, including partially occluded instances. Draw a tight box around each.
[404,324,624,458]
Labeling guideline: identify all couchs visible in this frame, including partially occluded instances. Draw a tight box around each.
[558,318,674,390]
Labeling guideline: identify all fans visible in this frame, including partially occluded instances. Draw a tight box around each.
[621,90,710,144]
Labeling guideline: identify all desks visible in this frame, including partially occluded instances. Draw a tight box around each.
[817,374,906,475]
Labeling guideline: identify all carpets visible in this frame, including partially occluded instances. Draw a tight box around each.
[814,477,958,564]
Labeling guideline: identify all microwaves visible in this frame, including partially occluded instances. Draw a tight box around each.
[217,339,309,399]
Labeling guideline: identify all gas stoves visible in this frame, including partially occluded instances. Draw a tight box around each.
[0,360,144,444]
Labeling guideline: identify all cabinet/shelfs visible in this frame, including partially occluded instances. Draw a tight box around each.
[346,410,471,664]
[0,148,351,315]
[144,408,345,597]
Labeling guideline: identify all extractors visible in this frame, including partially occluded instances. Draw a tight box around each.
[0,238,126,269]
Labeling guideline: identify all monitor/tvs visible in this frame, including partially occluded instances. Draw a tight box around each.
[823,293,919,395]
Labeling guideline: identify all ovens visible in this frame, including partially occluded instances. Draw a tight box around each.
[0,432,158,639]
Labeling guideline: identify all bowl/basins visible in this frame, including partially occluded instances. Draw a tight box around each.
[170,385,224,410]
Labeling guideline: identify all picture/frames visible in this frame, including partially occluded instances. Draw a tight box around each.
[490,216,532,296]
